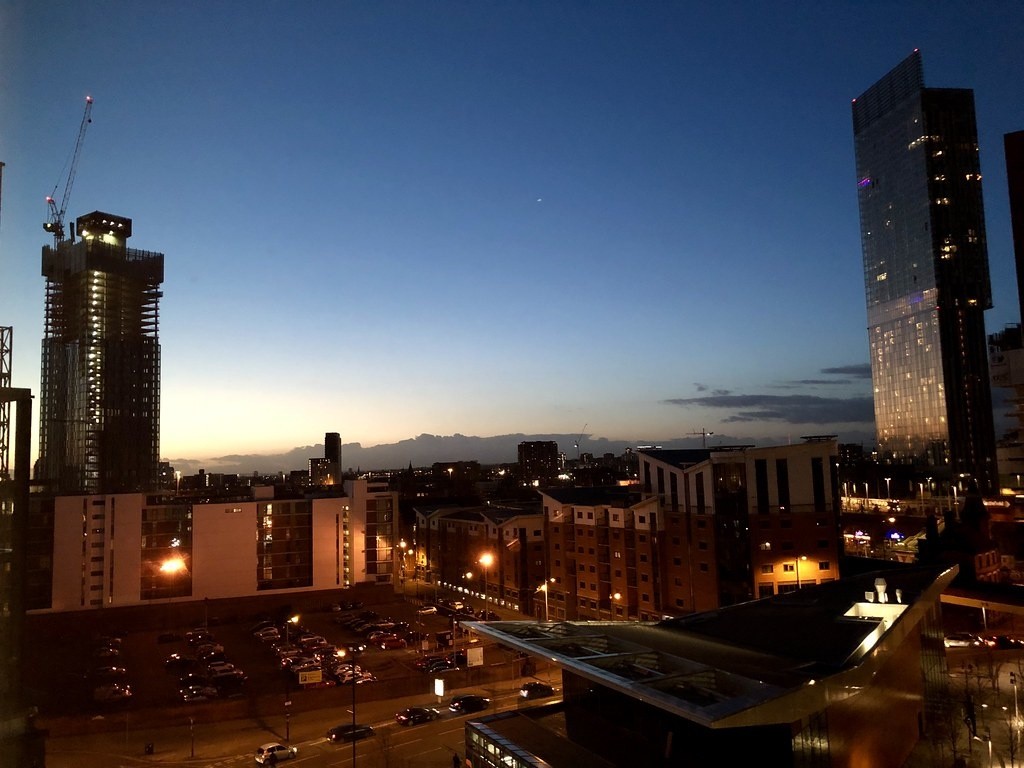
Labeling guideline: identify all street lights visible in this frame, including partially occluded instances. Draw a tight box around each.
[479,550,494,621]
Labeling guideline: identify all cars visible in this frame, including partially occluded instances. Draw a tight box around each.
[161,627,247,704]
[462,605,474,614]
[476,609,497,620]
[93,666,127,679]
[91,633,122,659]
[431,597,445,604]
[416,650,468,674]
[396,707,440,727]
[327,725,374,745]
[248,598,409,689]
[406,630,430,642]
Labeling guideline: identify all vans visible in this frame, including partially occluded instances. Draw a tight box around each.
[92,683,134,705]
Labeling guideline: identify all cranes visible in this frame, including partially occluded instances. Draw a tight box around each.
[43,97,93,245]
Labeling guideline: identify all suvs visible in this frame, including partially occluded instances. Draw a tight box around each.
[520,683,553,698]
[255,742,297,765]
[448,601,464,610]
[449,695,489,713]
[417,606,438,616]
